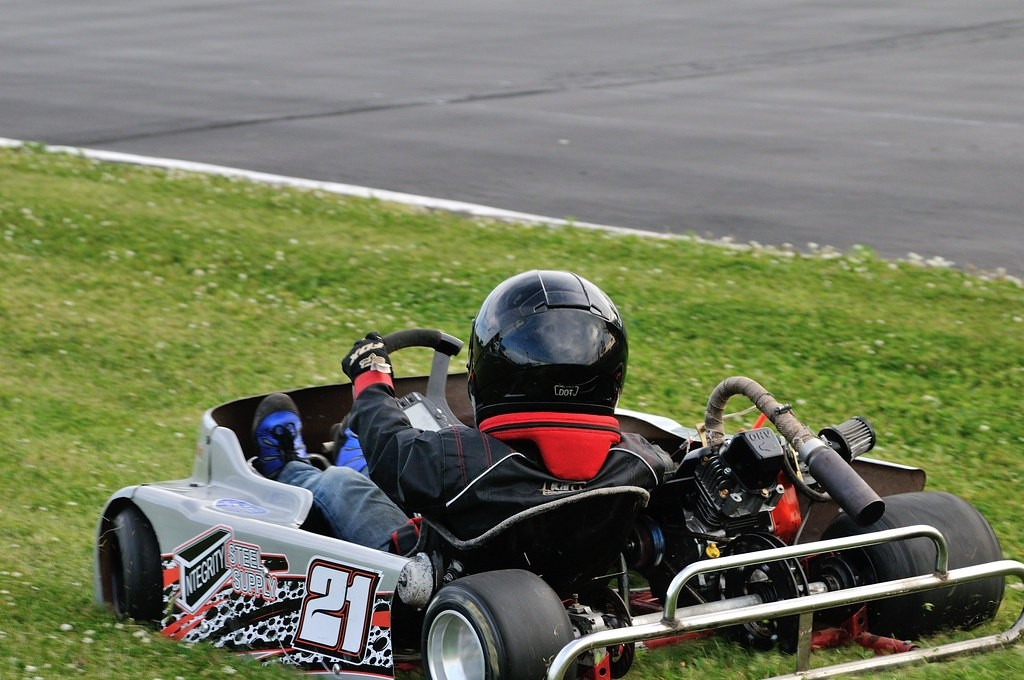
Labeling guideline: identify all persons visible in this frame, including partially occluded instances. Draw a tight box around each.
[252,269,674,553]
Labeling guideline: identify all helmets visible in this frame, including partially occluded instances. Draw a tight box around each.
[467,269,628,418]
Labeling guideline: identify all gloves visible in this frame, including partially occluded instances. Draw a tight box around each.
[341,332,394,383]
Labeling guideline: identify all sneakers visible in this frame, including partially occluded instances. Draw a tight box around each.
[251,393,312,480]
[333,414,367,473]
